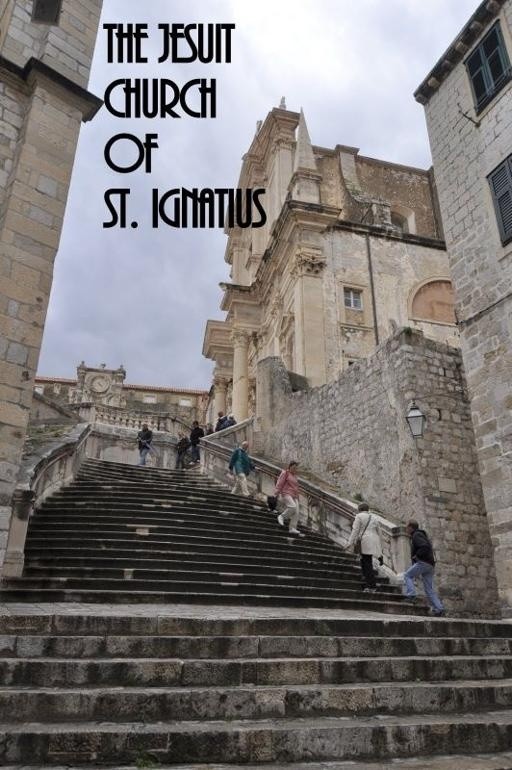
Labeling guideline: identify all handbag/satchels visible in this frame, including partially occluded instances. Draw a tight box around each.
[353,540,362,554]
[266,496,278,512]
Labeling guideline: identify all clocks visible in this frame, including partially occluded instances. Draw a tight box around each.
[88,375,110,393]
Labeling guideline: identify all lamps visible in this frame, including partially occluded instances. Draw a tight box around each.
[406,397,427,439]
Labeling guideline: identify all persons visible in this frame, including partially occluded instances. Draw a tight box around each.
[342,503,385,593]
[228,414,236,426]
[187,420,204,465]
[272,460,301,534]
[228,440,262,499]
[173,431,192,472]
[402,519,445,618]
[206,423,214,435]
[135,423,154,467]
[215,411,231,432]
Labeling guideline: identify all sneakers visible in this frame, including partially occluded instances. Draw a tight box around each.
[288,528,300,535]
[428,607,444,614]
[403,595,419,604]
[277,515,285,525]
[364,587,378,594]
